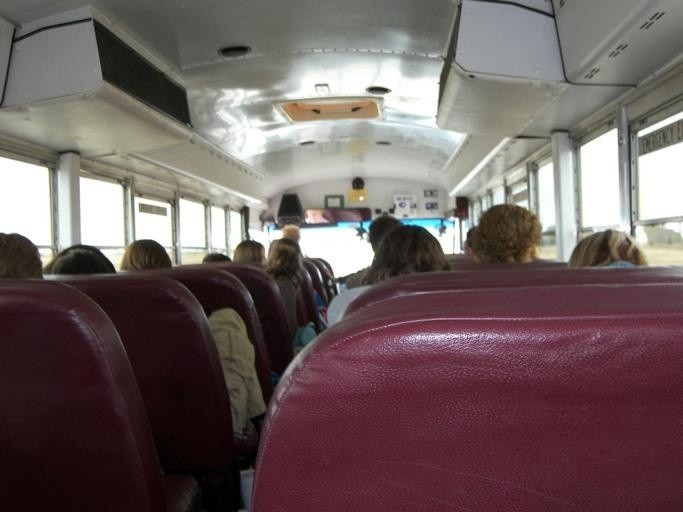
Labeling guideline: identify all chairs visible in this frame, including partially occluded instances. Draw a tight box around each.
[306,208,368,225]
[0,253,682,512]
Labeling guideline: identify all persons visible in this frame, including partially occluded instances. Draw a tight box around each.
[472,203,544,268]
[568,229,647,269]
[368,216,402,258]
[119,225,325,389]
[460,228,486,266]
[0,231,40,281]
[326,223,450,328]
[41,244,266,441]
